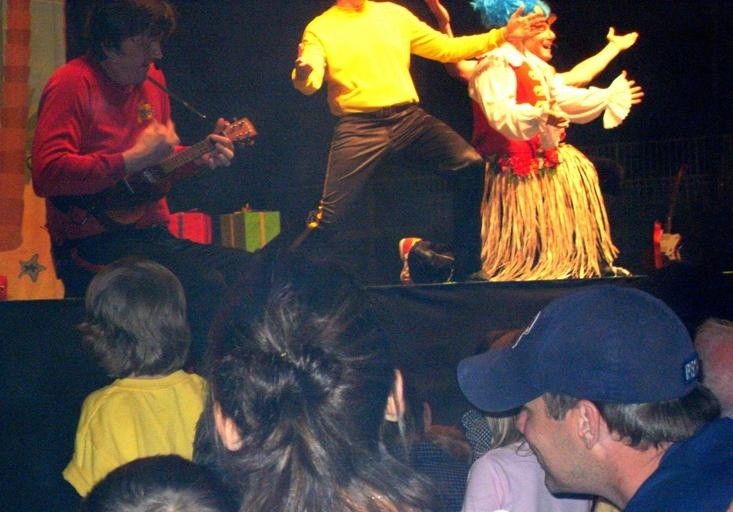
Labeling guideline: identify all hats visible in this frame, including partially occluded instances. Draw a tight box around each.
[456,284,700,414]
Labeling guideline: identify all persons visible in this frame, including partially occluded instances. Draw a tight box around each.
[455,285,732,512]
[200,254,451,510]
[291,0,548,282]
[377,224,733,512]
[424,0,640,88]
[59,255,217,496]
[30,0,263,299]
[468,0,644,282]
[80,455,237,511]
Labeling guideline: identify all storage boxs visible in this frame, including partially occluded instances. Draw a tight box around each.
[219,210,281,255]
[169,207,213,245]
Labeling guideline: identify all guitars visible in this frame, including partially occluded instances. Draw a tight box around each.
[86,116,257,229]
[652,162,685,268]
